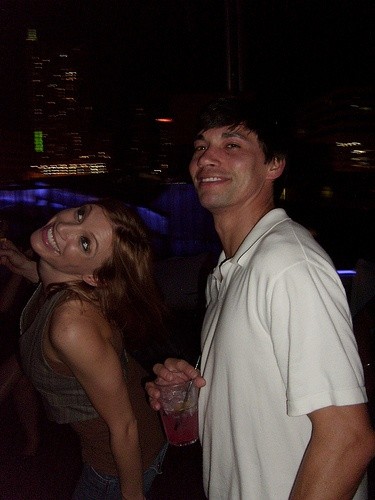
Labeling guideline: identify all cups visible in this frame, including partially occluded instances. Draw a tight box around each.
[155,368,199,447]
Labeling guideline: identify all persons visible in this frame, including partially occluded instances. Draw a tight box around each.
[134,88,374,500]
[0,197,173,500]
